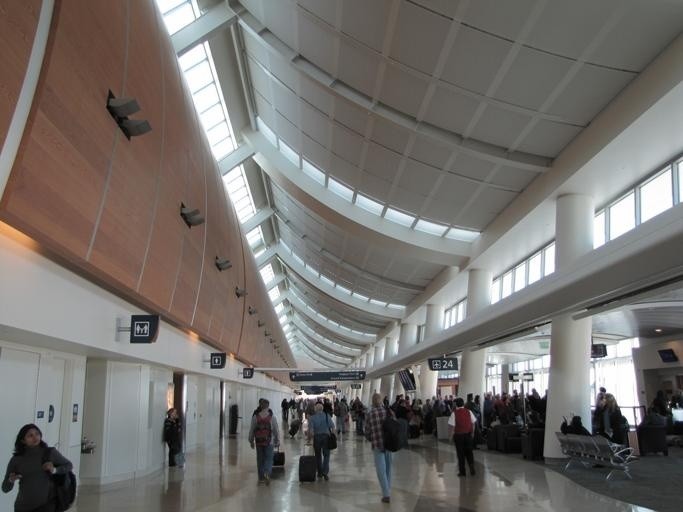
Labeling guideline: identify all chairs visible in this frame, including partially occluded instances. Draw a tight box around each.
[553,430,636,481]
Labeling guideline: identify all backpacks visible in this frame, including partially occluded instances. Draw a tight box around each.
[254,411,272,446]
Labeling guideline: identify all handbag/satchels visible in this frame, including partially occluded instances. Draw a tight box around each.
[382,417,407,452]
[325,431,337,450]
[50,472,76,510]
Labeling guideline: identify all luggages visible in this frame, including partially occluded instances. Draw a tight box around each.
[299,444,317,484]
[273,444,285,466]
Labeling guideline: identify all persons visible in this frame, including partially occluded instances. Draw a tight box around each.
[1,422,73,511]
[447,396,478,479]
[383,394,456,435]
[282,397,314,437]
[592,386,629,455]
[560,415,594,458]
[142,324,147,333]
[644,389,683,425]
[464,392,482,450]
[162,408,183,468]
[245,399,280,487]
[323,395,367,434]
[135,324,140,334]
[362,392,397,504]
[253,399,273,416]
[306,399,337,483]
[483,388,547,430]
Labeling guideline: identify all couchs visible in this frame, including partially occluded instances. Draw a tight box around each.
[483,421,543,460]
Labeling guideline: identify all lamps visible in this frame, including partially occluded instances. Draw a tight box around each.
[105,90,140,121]
[179,201,204,228]
[215,255,232,272]
[235,286,248,298]
[116,118,152,140]
[249,307,291,368]
